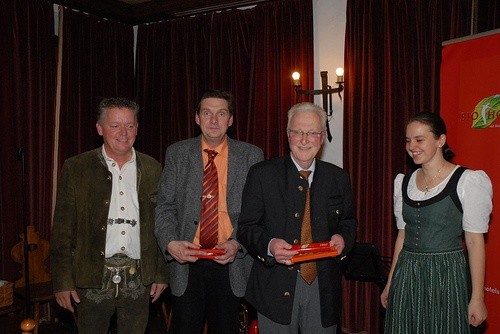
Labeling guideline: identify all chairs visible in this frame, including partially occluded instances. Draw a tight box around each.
[10,224,55,334]
[342,242,392,334]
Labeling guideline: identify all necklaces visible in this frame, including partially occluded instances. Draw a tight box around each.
[422,169,445,192]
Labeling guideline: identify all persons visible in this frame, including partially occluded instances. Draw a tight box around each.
[380,111,493,334]
[154,89,263,334]
[50,98,169,334]
[236,103,358,334]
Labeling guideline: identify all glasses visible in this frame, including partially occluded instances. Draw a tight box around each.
[287,127,324,140]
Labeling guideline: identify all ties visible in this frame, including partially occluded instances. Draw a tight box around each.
[298,170,318,285]
[199,148,220,251]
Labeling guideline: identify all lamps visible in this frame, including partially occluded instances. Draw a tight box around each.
[291,67,345,142]
